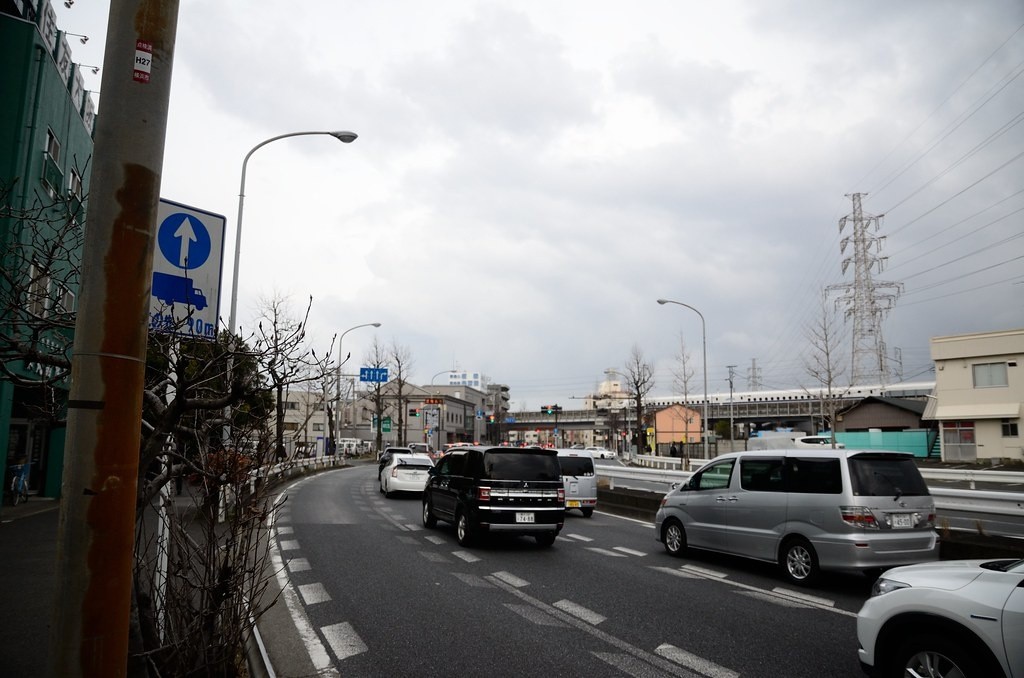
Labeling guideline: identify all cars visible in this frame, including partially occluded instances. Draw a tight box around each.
[856,557,1024,678]
[336,436,587,476]
[584,446,616,461]
[237,439,318,462]
[380,452,436,499]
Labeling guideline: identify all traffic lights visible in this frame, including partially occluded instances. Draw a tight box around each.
[488,415,496,423]
[546,405,553,415]
[623,430,627,436]
[415,409,421,417]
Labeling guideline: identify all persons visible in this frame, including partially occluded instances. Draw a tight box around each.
[670,444,678,457]
[647,444,652,455]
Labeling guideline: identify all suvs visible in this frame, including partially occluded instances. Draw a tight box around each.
[653,446,942,590]
[423,445,566,549]
[793,435,845,451]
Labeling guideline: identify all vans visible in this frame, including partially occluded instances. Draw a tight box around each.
[545,448,598,518]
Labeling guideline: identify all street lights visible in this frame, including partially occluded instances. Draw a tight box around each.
[656,297,709,462]
[334,323,382,456]
[604,371,632,463]
[217,131,358,524]
[430,370,458,449]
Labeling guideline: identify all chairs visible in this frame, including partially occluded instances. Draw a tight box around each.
[751,472,772,489]
